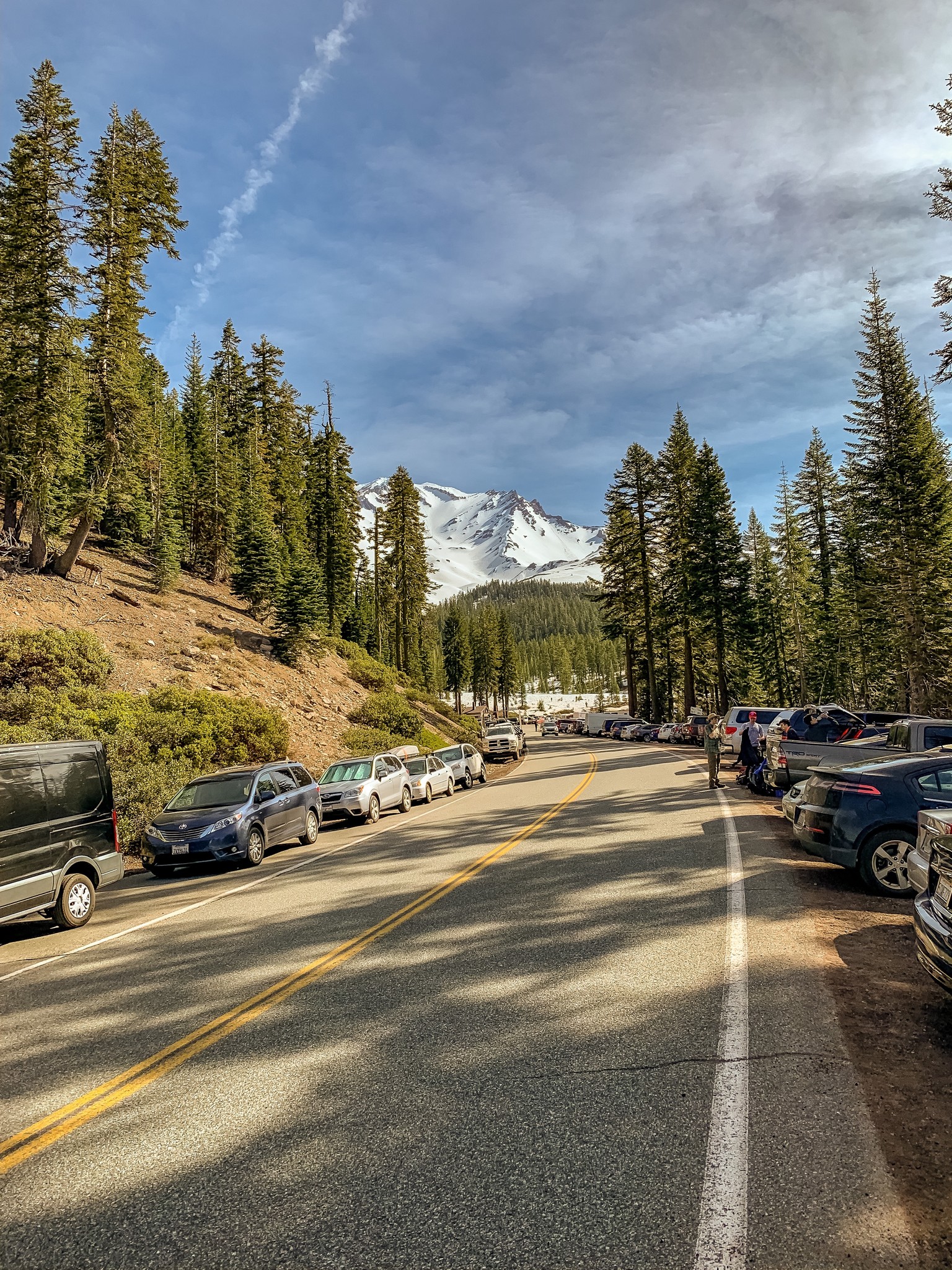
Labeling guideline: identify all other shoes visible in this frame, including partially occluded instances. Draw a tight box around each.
[709,784,718,789]
[715,783,726,787]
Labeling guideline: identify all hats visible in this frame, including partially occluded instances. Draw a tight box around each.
[749,712,757,718]
[706,713,720,721]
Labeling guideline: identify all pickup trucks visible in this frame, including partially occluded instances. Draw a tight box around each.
[762,704,952,825]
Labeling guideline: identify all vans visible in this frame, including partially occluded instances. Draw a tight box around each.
[583,712,635,738]
[0,740,125,931]
[719,706,784,755]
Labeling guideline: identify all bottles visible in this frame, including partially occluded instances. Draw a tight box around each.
[816,710,829,720]
[776,790,783,797]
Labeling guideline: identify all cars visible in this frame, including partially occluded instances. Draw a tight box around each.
[790,746,952,893]
[475,715,584,760]
[905,805,951,892]
[386,744,455,804]
[912,834,951,1000]
[601,715,710,745]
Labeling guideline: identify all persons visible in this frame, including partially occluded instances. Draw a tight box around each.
[739,711,764,776]
[703,713,726,790]
[779,719,800,741]
[804,713,839,743]
[534,720,538,732]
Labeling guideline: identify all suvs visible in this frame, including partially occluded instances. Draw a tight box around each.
[139,760,323,879]
[540,722,559,736]
[431,743,488,790]
[317,751,414,825]
